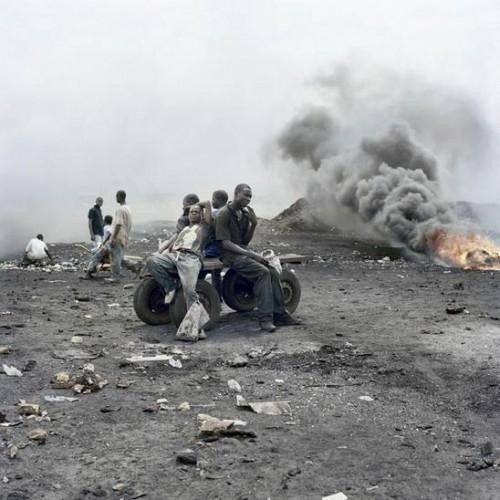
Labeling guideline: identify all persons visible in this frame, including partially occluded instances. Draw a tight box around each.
[146,201,213,342]
[24,232,52,266]
[198,189,229,302]
[177,194,200,235]
[88,198,105,252]
[80,189,133,281]
[214,183,304,334]
[103,216,113,240]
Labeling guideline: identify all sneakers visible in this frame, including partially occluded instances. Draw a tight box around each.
[259,321,276,332]
[273,310,302,325]
[198,329,207,339]
[164,280,180,304]
